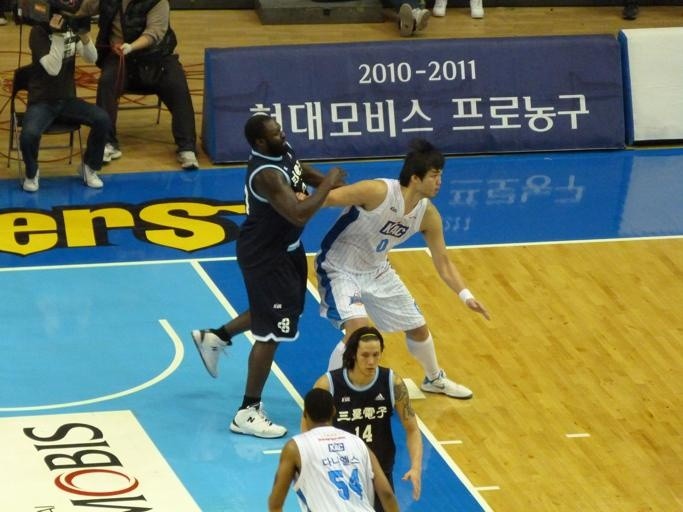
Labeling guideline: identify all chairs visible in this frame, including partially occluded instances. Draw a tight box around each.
[102,143,122,161]
[192,328,231,377]
[22,168,40,193]
[422,370,474,398]
[471,0,484,19]
[178,150,199,171]
[624,5,639,20]
[434,0,447,18]
[399,3,413,37]
[230,401,287,438]
[414,8,431,31]
[78,162,102,188]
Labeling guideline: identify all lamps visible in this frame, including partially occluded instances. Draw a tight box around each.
[3,60,77,168]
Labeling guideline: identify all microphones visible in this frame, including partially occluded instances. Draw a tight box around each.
[114,87,166,125]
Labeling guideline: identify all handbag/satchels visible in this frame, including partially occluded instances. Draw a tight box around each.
[457,288,475,306]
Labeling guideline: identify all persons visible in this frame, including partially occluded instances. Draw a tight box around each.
[265,387,401,512]
[16,0,111,196]
[431,0,485,20]
[619,0,640,21]
[394,0,430,37]
[297,327,423,511]
[93,0,199,169]
[187,113,349,440]
[293,141,492,401]
